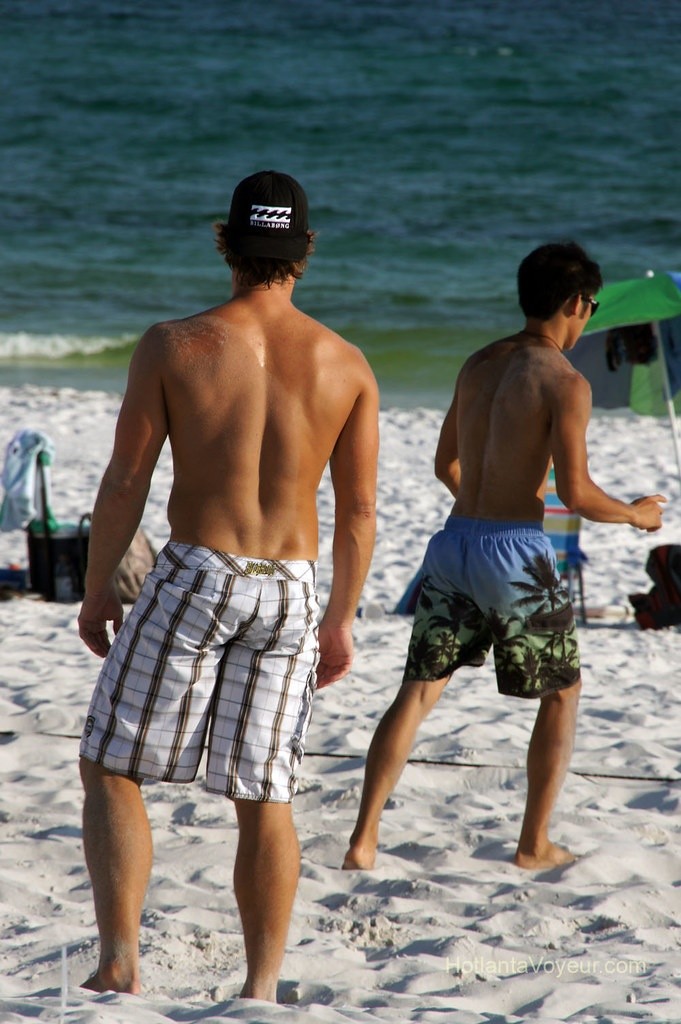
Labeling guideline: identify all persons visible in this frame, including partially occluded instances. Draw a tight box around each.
[342,240,669,872]
[76,167,381,1003]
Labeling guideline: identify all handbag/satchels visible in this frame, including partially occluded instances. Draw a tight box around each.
[116,527,156,601]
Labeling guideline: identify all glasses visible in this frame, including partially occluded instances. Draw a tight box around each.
[575,294,598,315]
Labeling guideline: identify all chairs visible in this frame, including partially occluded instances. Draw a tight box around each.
[538,459,587,626]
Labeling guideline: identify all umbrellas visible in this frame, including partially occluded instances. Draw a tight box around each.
[579,271,680,466]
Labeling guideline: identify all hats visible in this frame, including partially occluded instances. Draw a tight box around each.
[223,171,309,260]
